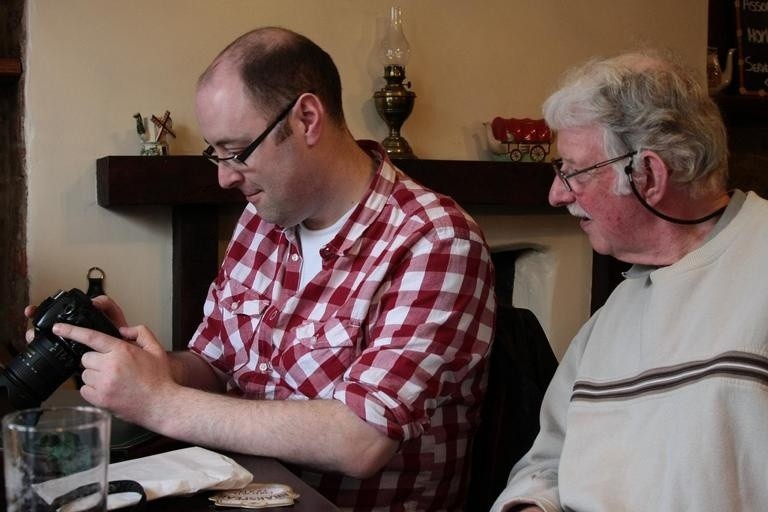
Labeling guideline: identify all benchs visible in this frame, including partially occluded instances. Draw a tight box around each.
[95,155,634,351]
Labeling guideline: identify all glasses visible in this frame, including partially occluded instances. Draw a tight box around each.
[201,85,320,172]
[547,140,641,196]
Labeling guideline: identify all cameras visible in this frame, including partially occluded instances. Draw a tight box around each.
[0,287,122,421]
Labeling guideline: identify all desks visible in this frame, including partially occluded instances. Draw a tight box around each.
[0,390,342,511]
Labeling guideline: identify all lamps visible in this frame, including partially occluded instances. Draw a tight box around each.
[373,6,418,160]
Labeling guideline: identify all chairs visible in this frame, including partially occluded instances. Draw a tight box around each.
[472,306,560,511]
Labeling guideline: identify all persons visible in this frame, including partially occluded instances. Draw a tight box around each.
[486,45,766,510]
[23,25,498,510]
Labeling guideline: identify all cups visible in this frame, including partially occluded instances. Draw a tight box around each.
[1,406,112,512]
